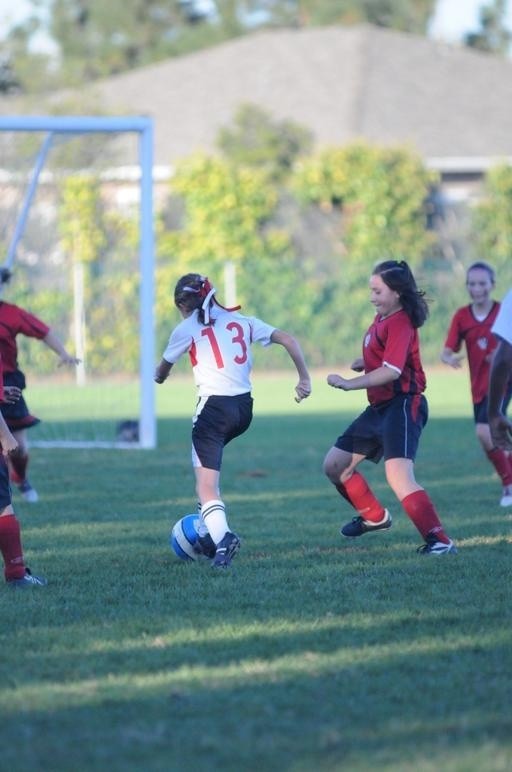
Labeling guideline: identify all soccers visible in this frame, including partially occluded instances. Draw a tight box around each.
[172,515,205,562]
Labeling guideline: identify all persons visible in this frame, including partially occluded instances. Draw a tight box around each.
[439,261,512,509]
[489,289,512,452]
[154,273,312,569]
[0,264,81,503]
[323,258,458,556]
[1,324,47,586]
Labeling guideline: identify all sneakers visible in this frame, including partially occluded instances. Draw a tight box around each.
[340,507,392,538]
[10,475,37,501]
[195,532,241,567]
[501,484,512,507]
[417,539,457,555]
[7,567,47,587]
